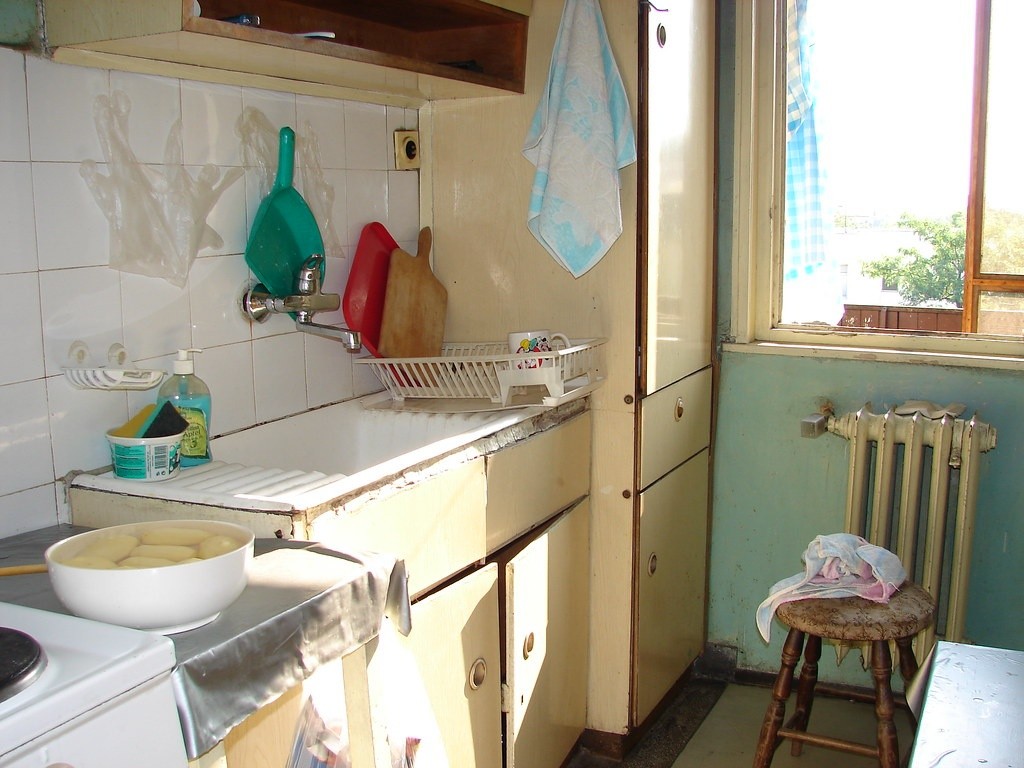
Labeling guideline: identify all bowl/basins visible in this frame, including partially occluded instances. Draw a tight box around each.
[44,518,255,636]
[105,431,186,482]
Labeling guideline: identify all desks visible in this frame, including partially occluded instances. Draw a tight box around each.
[0,523,412,768]
[905,641,1024,768]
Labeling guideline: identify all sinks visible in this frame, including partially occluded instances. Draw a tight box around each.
[206,406,499,479]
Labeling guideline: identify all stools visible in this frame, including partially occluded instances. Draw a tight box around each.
[752,580,939,768]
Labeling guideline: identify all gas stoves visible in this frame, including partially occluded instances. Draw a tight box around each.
[0,601,177,756]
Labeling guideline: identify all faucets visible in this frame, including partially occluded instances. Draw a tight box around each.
[296,311,362,354]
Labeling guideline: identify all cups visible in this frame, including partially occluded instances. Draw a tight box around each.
[509,329,571,371]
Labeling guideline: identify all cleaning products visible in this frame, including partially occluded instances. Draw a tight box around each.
[155,345,214,469]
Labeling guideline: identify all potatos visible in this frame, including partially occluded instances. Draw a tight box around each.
[73,527,241,569]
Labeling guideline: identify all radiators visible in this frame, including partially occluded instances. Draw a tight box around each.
[826,401,997,678]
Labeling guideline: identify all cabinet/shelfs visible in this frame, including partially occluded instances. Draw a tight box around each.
[418,0,718,763]
[36,0,532,108]
[302,408,591,768]
